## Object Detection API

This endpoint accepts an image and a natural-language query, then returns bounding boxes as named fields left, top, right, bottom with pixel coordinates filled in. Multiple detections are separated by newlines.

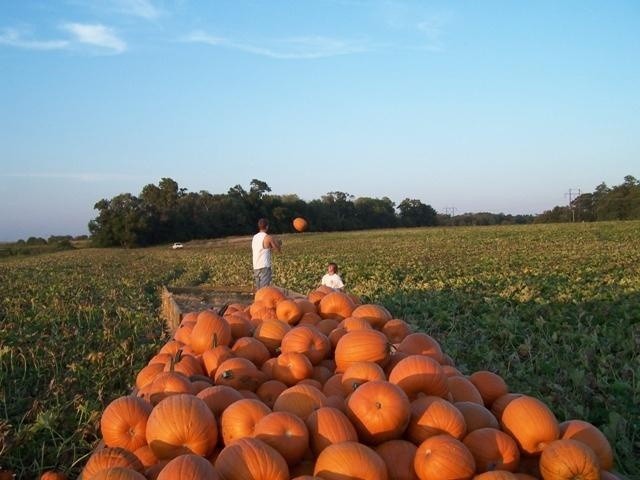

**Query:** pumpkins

left=81, top=285, right=618, bottom=480
left=41, top=468, right=68, bottom=480
left=292, top=217, right=308, bottom=232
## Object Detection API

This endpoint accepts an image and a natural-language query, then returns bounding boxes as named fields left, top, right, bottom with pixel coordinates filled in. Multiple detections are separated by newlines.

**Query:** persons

left=252, top=217, right=281, bottom=289
left=322, top=262, right=345, bottom=293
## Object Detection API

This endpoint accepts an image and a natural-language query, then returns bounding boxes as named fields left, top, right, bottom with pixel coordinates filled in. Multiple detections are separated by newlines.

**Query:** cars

left=172, top=242, right=183, bottom=250
